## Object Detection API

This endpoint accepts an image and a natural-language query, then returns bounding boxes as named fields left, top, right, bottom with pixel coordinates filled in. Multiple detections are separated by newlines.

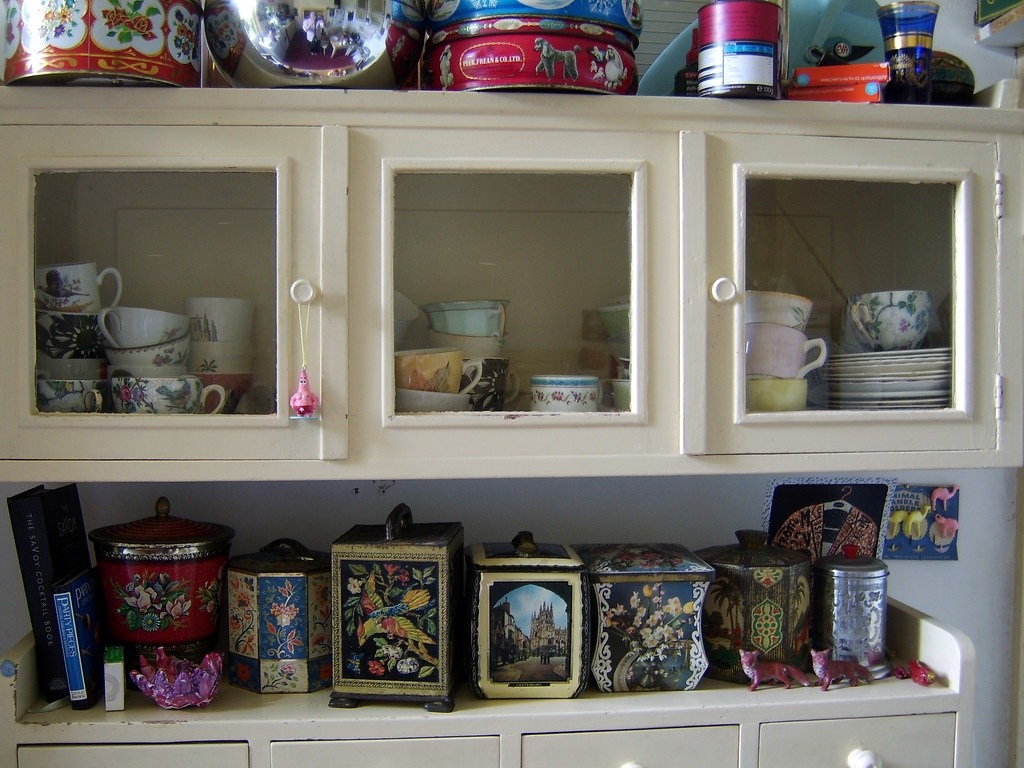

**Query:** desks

left=325, top=27, right=355, bottom=58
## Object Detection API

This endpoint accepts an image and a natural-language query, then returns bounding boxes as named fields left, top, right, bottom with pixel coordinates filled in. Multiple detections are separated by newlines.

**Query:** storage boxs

left=786, top=62, right=891, bottom=103
left=102, top=646, right=125, bottom=711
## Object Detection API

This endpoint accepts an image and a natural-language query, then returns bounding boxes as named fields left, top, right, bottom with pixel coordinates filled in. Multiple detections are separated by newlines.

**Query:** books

left=6, top=482, right=106, bottom=710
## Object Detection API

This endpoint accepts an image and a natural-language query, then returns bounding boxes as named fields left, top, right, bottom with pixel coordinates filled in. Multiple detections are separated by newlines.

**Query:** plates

left=809, top=348, right=952, bottom=409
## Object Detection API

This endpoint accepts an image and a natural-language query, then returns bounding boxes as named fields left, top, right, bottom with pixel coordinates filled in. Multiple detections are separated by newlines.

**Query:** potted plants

left=264, top=7, right=282, bottom=39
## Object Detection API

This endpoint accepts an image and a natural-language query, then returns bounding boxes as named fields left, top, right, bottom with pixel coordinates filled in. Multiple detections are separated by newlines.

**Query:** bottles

left=89, top=495, right=234, bottom=691
left=694, top=528, right=814, bottom=682
left=819, top=545, right=892, bottom=679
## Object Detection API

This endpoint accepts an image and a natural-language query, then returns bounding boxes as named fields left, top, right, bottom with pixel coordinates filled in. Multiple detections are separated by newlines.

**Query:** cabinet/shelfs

left=0, top=595, right=977, bottom=768
left=0, top=86, right=1024, bottom=482
left=287, top=21, right=296, bottom=41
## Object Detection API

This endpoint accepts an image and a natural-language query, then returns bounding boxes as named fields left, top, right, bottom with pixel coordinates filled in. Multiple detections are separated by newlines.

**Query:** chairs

left=320, top=40, right=329, bottom=55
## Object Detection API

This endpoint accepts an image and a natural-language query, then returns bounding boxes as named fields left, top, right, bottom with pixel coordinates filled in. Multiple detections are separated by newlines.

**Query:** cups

left=529, top=294, right=631, bottom=413
left=847, top=290, right=933, bottom=351
left=393, top=298, right=520, bottom=411
left=878, top=3, right=941, bottom=103
left=745, top=289, right=828, bottom=411
left=36, top=260, right=256, bottom=414
